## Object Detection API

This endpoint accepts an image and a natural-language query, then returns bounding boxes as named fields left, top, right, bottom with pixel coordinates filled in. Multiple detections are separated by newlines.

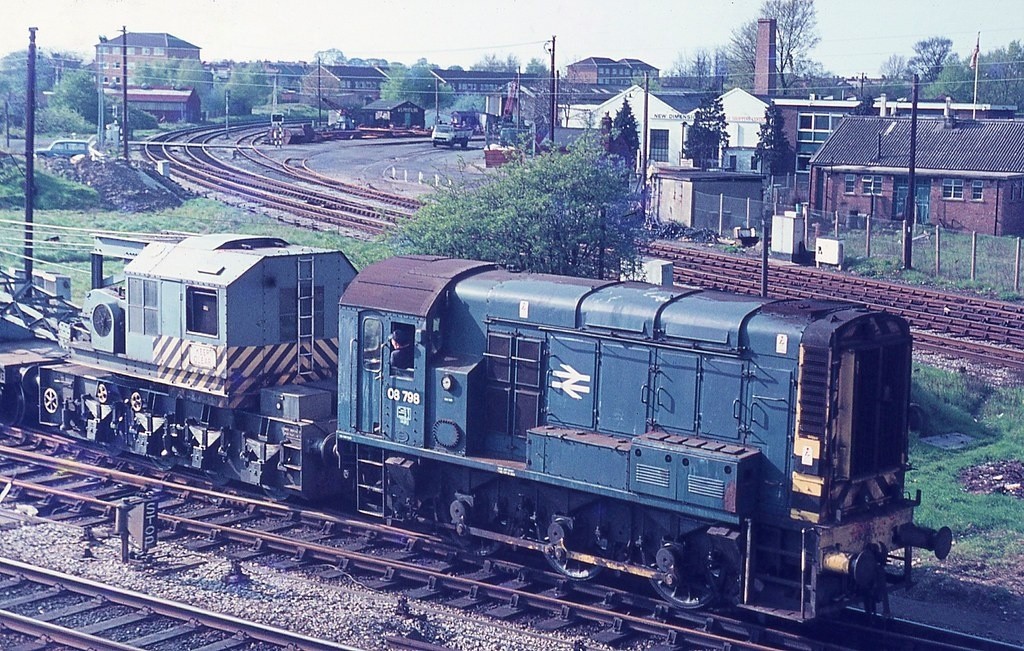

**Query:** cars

left=34, top=139, right=91, bottom=161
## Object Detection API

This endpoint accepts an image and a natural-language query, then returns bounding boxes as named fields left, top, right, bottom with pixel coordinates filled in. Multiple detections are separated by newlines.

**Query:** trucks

left=431, top=124, right=476, bottom=150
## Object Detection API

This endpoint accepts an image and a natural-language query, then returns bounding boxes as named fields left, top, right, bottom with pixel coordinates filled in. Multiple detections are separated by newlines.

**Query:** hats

left=388, top=330, right=409, bottom=344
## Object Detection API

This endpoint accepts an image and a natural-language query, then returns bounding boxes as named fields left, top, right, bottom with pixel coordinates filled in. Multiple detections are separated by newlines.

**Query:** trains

left=2, top=227, right=951, bottom=633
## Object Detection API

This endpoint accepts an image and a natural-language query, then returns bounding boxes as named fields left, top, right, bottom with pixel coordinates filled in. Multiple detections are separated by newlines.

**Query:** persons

left=387, top=329, right=415, bottom=368
